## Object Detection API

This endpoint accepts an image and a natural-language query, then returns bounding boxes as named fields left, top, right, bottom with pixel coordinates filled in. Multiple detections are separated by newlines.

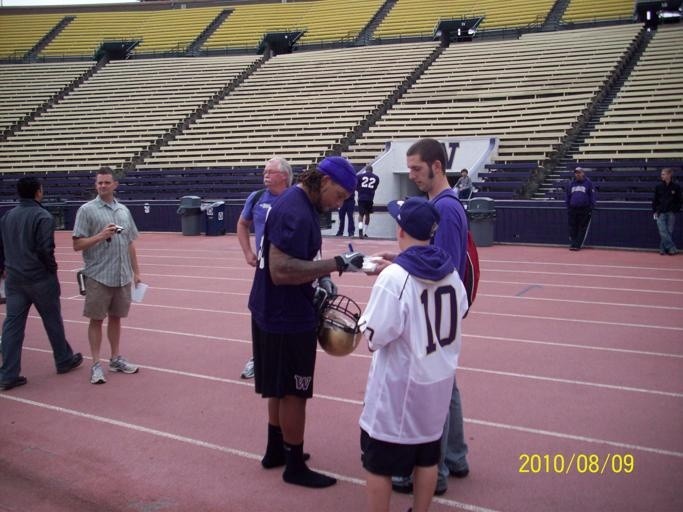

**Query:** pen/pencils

left=348, top=244, right=354, bottom=252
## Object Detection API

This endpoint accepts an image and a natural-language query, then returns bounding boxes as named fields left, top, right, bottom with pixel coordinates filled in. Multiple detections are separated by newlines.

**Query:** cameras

left=113, top=225, right=124, bottom=234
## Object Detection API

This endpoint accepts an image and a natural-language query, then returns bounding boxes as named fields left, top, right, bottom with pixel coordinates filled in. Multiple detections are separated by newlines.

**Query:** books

left=358, top=255, right=384, bottom=273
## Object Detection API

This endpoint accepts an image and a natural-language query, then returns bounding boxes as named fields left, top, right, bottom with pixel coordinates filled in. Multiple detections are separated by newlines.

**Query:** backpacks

left=432, top=194, right=480, bottom=319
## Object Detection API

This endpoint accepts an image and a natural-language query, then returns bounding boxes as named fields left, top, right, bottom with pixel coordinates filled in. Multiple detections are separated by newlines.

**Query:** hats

left=574, top=167, right=583, bottom=173
left=315, top=155, right=358, bottom=194
left=387, top=196, right=440, bottom=240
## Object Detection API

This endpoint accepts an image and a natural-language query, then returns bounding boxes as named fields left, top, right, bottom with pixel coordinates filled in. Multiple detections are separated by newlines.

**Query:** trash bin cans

left=180, top=196, right=200, bottom=235
left=204, top=201, right=226, bottom=236
left=468, top=199, right=497, bottom=248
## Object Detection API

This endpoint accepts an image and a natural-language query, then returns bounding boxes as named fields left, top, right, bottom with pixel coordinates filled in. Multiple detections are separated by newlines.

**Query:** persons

left=0, top=176, right=84, bottom=391
left=563, top=166, right=598, bottom=251
left=363, top=138, right=470, bottom=496
left=652, top=167, right=683, bottom=255
left=355, top=195, right=470, bottom=512
left=72, top=166, right=144, bottom=385
left=334, top=191, right=357, bottom=238
left=239, top=156, right=294, bottom=380
left=455, top=168, right=474, bottom=211
left=354, top=164, right=379, bottom=239
left=246, top=155, right=364, bottom=489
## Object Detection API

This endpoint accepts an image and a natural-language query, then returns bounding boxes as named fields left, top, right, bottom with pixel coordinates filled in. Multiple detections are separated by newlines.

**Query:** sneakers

left=660, top=250, right=665, bottom=255
left=336, top=232, right=343, bottom=236
left=349, top=232, right=353, bottom=239
left=570, top=242, right=579, bottom=250
left=668, top=250, right=679, bottom=254
left=240, top=356, right=255, bottom=379
left=89, top=361, right=107, bottom=384
left=108, top=355, right=139, bottom=374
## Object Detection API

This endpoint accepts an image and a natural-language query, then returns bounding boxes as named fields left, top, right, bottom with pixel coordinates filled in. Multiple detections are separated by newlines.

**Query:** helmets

left=318, top=294, right=362, bottom=357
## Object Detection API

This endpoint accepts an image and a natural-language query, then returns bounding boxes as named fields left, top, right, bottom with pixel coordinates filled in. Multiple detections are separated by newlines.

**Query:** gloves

left=334, top=251, right=364, bottom=277
left=319, top=274, right=337, bottom=301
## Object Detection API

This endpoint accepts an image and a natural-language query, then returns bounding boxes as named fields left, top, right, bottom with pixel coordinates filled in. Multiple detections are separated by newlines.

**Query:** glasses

left=263, top=170, right=279, bottom=174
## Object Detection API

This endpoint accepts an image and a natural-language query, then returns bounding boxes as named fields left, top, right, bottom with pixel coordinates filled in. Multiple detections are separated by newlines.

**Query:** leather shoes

left=0, top=376, right=27, bottom=390
left=391, top=476, right=448, bottom=495
left=57, top=352, right=82, bottom=374
left=449, top=468, right=469, bottom=477
